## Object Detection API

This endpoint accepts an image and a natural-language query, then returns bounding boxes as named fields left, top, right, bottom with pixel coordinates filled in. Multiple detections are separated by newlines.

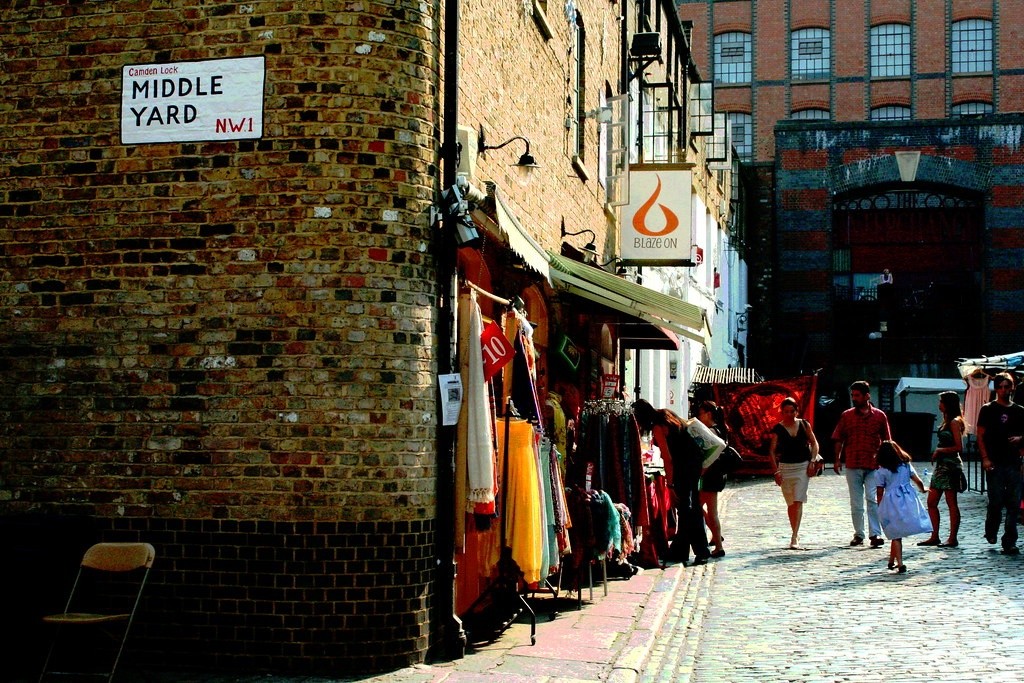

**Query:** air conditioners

left=630, top=32, right=661, bottom=56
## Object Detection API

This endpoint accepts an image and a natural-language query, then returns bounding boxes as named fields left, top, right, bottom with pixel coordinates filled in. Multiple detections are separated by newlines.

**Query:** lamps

left=481, top=137, right=541, bottom=187
left=561, top=221, right=600, bottom=264
left=594, top=254, right=631, bottom=278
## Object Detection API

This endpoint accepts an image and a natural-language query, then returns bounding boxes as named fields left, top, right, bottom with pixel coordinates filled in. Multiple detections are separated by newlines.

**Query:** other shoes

left=1000, top=540, right=1020, bottom=556
left=984, top=534, right=997, bottom=545
left=898, top=565, right=907, bottom=574
left=708, top=536, right=724, bottom=546
left=789, top=535, right=800, bottom=550
left=938, top=539, right=959, bottom=547
left=694, top=554, right=707, bottom=564
left=888, top=562, right=895, bottom=570
left=917, top=538, right=941, bottom=547
left=850, top=536, right=864, bottom=546
left=710, top=550, right=725, bottom=558
left=869, top=535, right=885, bottom=547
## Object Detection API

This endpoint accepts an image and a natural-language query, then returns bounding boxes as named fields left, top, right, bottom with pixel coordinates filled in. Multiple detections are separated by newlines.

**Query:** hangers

left=962, top=363, right=994, bottom=380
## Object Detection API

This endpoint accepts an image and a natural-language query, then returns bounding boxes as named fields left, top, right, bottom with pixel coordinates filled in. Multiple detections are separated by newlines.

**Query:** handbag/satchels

left=806, top=454, right=825, bottom=478
left=705, top=426, right=743, bottom=478
left=679, top=417, right=728, bottom=468
left=953, top=470, right=967, bottom=493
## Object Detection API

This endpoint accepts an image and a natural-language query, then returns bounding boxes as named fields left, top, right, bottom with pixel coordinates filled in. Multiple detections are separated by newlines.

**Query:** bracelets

left=774, top=470, right=781, bottom=475
left=810, top=458, right=816, bottom=463
left=666, top=484, right=675, bottom=489
left=982, top=454, right=988, bottom=458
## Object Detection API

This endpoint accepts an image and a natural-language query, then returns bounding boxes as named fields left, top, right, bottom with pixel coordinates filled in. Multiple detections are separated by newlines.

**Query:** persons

left=770, top=397, right=819, bottom=550
left=916, top=391, right=967, bottom=547
left=976, top=372, right=1024, bottom=554
left=630, top=399, right=727, bottom=566
left=832, top=381, right=892, bottom=547
left=873, top=441, right=930, bottom=572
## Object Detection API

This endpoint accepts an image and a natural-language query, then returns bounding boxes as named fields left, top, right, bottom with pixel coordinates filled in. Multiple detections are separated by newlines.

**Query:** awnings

left=493, top=184, right=553, bottom=289
left=546, top=250, right=712, bottom=347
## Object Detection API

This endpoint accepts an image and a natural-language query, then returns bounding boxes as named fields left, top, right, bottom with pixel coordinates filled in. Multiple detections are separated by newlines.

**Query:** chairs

left=41, top=542, right=156, bottom=683
